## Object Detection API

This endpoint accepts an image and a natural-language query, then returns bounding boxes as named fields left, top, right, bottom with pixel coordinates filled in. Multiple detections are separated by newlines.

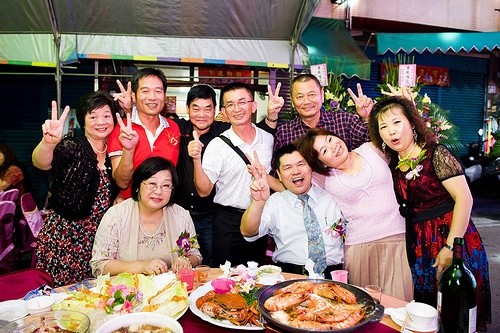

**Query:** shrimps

left=265, top=280, right=366, bottom=330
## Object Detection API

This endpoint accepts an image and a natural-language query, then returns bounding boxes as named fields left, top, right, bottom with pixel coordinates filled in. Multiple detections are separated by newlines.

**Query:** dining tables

left=52, top=268, right=438, bottom=333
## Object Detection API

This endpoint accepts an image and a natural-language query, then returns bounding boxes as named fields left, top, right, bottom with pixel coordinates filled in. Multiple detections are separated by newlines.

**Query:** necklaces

left=396, top=140, right=418, bottom=162
left=87, top=138, right=108, bottom=152
left=139, top=216, right=164, bottom=237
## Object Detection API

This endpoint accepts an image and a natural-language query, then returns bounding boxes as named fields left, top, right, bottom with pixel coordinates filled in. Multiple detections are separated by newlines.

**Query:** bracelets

left=266, top=116, right=279, bottom=123
left=444, top=243, right=454, bottom=251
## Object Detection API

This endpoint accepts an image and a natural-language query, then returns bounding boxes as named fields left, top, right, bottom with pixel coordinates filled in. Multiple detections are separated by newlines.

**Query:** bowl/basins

left=90, top=311, right=184, bottom=333
left=25, top=295, right=54, bottom=313
left=256, top=278, right=385, bottom=332
left=405, top=301, right=437, bottom=326
left=0, top=310, right=91, bottom=333
left=211, top=279, right=237, bottom=293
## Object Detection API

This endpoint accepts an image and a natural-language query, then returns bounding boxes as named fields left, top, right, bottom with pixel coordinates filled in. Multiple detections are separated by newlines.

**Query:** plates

left=391, top=307, right=439, bottom=332
left=0, top=299, right=28, bottom=324
left=57, top=269, right=271, bottom=333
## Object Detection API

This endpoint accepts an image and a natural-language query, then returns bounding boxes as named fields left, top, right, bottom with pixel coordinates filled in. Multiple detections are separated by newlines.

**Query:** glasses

left=141, top=179, right=174, bottom=192
left=223, top=100, right=254, bottom=107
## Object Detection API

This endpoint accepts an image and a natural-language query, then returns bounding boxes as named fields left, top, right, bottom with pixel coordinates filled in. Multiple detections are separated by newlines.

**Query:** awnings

left=368, top=26, right=500, bottom=60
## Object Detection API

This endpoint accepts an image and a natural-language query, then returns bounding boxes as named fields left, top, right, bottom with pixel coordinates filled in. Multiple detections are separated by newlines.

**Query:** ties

left=298, top=194, right=327, bottom=276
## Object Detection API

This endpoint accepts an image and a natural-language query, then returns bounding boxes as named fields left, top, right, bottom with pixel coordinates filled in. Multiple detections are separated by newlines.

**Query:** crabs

left=195, top=289, right=262, bottom=328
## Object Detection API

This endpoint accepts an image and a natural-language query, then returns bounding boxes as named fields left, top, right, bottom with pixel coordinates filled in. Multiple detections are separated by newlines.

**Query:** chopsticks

left=401, top=311, right=411, bottom=332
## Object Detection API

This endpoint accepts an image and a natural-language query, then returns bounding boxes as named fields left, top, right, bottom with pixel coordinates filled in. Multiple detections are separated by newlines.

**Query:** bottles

left=437, top=236, right=477, bottom=333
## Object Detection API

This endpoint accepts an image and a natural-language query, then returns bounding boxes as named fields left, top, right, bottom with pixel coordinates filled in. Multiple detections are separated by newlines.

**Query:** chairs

left=0, top=187, right=19, bottom=201
left=0, top=271, right=54, bottom=303
left=0, top=201, right=16, bottom=243
left=18, top=191, right=46, bottom=238
left=0, top=242, right=15, bottom=270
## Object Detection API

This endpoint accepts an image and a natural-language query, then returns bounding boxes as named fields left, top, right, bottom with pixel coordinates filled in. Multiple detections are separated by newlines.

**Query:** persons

left=369, top=94, right=492, bottom=332
left=247, top=82, right=416, bottom=301
left=106, top=67, right=182, bottom=202
left=269, top=73, right=375, bottom=185
left=88, top=155, right=203, bottom=275
left=187, top=82, right=274, bottom=267
left=239, top=142, right=347, bottom=281
left=110, top=78, right=286, bottom=265
left=166, top=112, right=179, bottom=120
left=0, top=143, right=27, bottom=221
left=31, top=90, right=117, bottom=286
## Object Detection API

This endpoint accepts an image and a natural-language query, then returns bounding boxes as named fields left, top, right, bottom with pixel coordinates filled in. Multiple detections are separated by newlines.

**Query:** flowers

left=173, top=231, right=203, bottom=258
left=372, top=68, right=464, bottom=152
left=395, top=148, right=428, bottom=180
left=324, top=215, right=349, bottom=241
left=93, top=281, right=140, bottom=315
left=320, top=71, right=355, bottom=111
left=210, top=260, right=260, bottom=295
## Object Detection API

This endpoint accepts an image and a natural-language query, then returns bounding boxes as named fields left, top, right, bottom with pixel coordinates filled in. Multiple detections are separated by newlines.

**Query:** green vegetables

left=237, top=286, right=261, bottom=305
left=104, top=290, right=136, bottom=314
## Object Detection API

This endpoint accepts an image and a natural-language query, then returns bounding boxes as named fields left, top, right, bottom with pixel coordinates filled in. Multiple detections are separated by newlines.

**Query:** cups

left=179, top=268, right=195, bottom=292
left=195, top=265, right=210, bottom=287
left=364, top=284, right=382, bottom=303
left=330, top=270, right=349, bottom=284
left=258, top=264, right=282, bottom=285
